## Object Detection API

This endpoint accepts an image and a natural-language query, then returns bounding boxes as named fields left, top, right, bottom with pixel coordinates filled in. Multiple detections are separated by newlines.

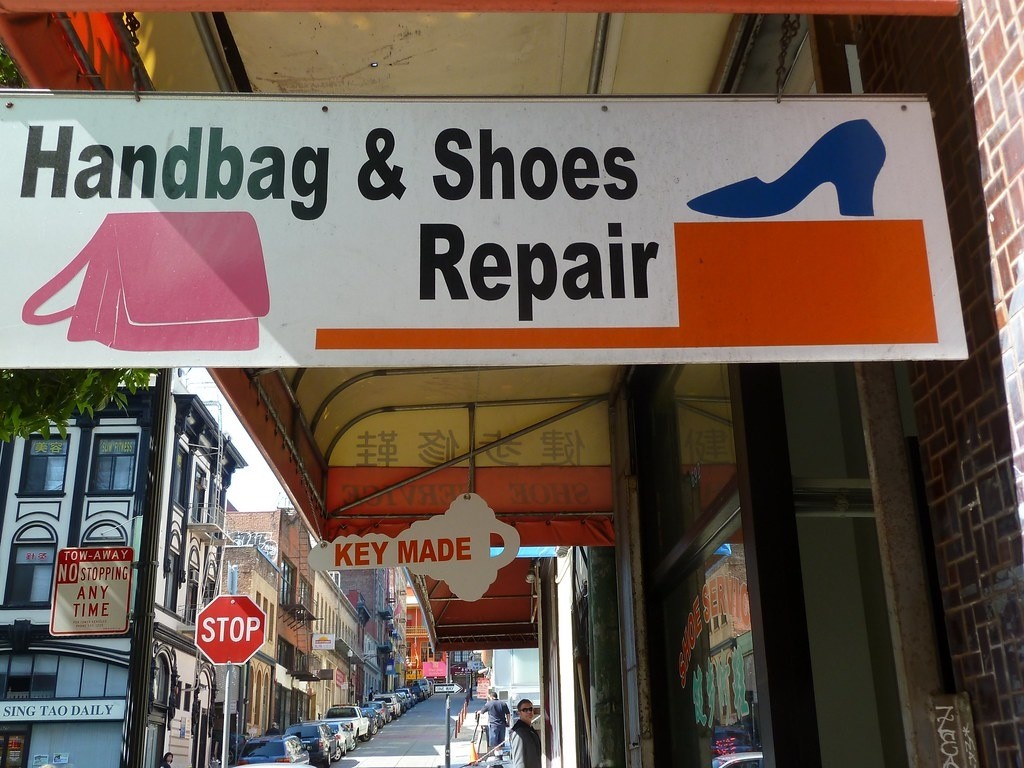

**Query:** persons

left=266, top=722, right=281, bottom=736
left=161, top=752, right=173, bottom=768
left=369, top=687, right=380, bottom=701
left=510, top=699, right=541, bottom=768
left=478, top=692, right=510, bottom=768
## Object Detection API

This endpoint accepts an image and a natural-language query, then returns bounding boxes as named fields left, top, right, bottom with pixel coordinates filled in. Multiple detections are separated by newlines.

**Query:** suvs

left=237, top=733, right=313, bottom=765
left=284, top=721, right=342, bottom=768
left=320, top=703, right=372, bottom=745
left=372, top=691, right=403, bottom=720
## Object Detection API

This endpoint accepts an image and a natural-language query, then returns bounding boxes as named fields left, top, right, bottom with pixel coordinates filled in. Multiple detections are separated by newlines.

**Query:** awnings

left=281, top=604, right=316, bottom=622
left=288, top=671, right=320, bottom=682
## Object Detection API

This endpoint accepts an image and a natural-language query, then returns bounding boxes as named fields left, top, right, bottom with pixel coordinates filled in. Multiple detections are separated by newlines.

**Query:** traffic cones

left=469, top=741, right=481, bottom=765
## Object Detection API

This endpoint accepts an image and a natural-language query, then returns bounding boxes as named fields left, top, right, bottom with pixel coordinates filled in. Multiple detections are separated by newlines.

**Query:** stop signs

left=194, top=595, right=267, bottom=665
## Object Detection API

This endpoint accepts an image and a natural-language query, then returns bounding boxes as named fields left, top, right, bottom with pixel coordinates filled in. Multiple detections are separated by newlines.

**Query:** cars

left=360, top=707, right=385, bottom=735
left=361, top=701, right=393, bottom=725
left=392, top=678, right=432, bottom=715
left=711, top=724, right=763, bottom=768
left=327, top=721, right=356, bottom=756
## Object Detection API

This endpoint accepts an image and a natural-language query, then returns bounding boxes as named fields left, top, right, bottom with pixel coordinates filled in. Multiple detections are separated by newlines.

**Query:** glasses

left=520, top=707, right=533, bottom=712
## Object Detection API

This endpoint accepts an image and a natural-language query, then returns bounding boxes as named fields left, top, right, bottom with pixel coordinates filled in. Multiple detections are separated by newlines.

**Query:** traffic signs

left=433, top=682, right=463, bottom=696
